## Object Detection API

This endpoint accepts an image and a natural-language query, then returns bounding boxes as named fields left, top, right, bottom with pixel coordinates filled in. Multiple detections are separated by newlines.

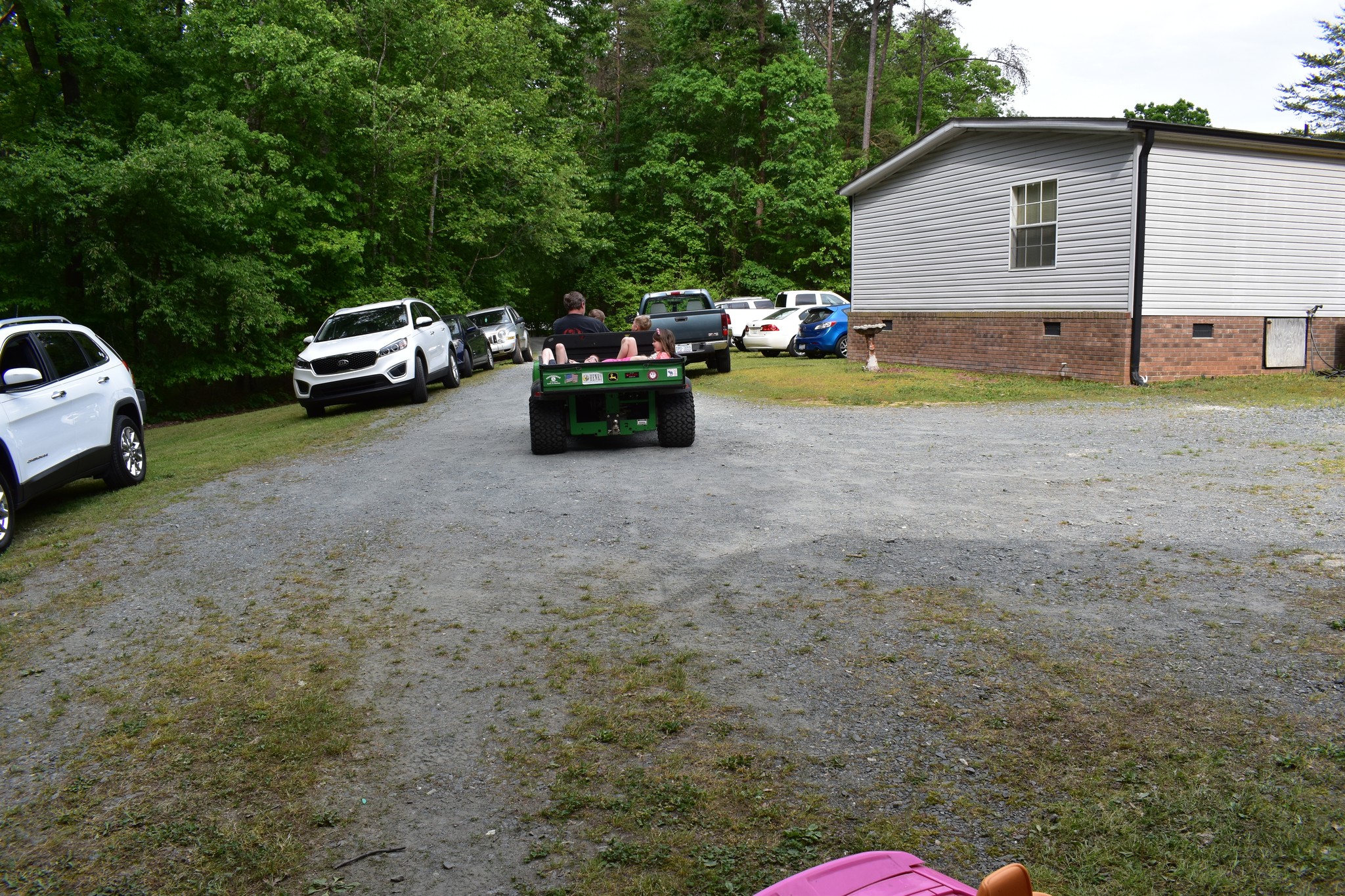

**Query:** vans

left=725, top=290, right=851, bottom=352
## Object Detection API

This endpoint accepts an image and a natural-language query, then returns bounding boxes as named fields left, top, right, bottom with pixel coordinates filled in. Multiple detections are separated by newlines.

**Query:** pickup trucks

left=625, top=288, right=731, bottom=373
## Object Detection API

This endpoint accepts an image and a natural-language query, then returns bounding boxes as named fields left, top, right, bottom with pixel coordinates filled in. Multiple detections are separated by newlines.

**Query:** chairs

left=0, top=344, right=38, bottom=382
left=686, top=299, right=703, bottom=311
left=650, top=302, right=667, bottom=314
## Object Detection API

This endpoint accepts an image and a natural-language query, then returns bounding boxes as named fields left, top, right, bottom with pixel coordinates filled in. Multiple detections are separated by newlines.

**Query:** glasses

left=585, top=357, right=598, bottom=362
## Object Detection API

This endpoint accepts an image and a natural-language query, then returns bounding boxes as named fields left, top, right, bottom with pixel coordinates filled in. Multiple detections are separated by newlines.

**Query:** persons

left=601, top=328, right=676, bottom=362
left=542, top=343, right=601, bottom=365
left=589, top=309, right=605, bottom=323
left=632, top=315, right=651, bottom=332
left=553, top=292, right=611, bottom=335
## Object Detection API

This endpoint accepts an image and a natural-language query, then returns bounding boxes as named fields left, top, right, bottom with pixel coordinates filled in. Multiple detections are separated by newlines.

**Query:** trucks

left=528, top=326, right=696, bottom=456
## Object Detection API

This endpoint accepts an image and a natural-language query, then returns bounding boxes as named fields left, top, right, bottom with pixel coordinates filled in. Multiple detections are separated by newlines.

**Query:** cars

left=793, top=303, right=851, bottom=359
left=440, top=314, right=494, bottom=378
left=742, top=307, right=814, bottom=357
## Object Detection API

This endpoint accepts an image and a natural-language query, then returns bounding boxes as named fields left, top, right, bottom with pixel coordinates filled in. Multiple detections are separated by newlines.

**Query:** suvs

left=292, top=298, right=460, bottom=418
left=713, top=296, right=775, bottom=347
left=466, top=305, right=533, bottom=365
left=0, top=314, right=148, bottom=555
